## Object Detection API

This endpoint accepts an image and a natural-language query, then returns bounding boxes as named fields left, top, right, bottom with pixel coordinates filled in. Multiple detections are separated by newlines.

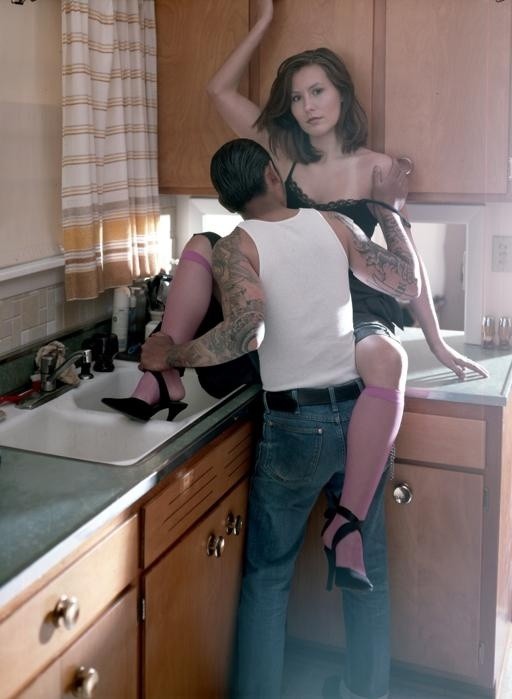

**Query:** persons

left=139, top=138, right=421, bottom=696
left=99, top=1, right=490, bottom=594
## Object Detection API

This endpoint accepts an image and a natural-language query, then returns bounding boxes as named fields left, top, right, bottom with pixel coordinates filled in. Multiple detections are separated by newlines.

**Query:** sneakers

left=321, top=675, right=344, bottom=699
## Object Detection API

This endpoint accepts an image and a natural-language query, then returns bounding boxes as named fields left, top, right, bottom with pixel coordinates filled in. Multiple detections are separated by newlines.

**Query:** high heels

left=100, top=368, right=190, bottom=423
left=318, top=503, right=375, bottom=594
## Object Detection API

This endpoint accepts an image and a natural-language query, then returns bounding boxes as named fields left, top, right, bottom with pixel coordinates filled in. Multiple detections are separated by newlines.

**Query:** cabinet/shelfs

left=290, top=405, right=510, bottom=695
left=0, top=508, right=140, bottom=699
left=144, top=418, right=262, bottom=695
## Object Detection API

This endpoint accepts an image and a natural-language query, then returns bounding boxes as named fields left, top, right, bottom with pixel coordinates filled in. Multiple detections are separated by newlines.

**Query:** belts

left=259, top=376, right=367, bottom=415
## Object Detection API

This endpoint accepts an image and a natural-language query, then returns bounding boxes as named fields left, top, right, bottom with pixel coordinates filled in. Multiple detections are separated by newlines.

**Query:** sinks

left=0, top=360, right=246, bottom=429
left=0, top=402, right=182, bottom=466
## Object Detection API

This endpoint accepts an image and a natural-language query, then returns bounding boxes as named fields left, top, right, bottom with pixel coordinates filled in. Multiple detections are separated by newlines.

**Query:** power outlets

left=490, top=233, right=512, bottom=273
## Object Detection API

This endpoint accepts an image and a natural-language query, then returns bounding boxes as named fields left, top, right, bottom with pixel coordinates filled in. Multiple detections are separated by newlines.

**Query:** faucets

left=39, top=350, right=91, bottom=391
